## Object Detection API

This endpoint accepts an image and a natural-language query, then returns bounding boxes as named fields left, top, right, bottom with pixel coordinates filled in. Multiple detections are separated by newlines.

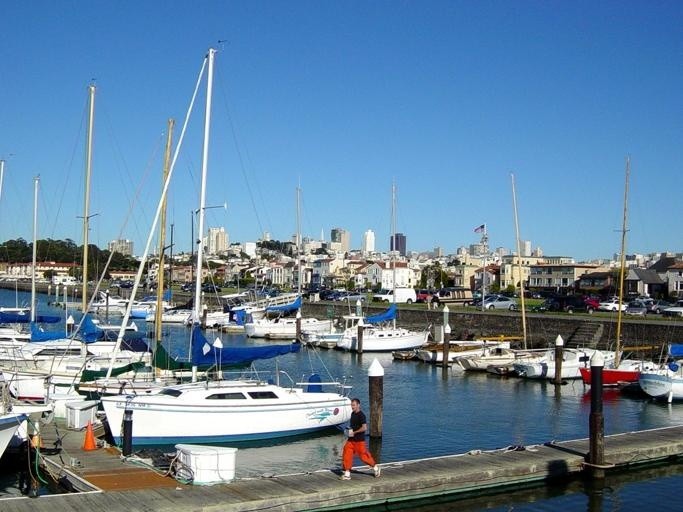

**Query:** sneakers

left=374, top=465, right=381, bottom=478
left=338, top=475, right=351, bottom=480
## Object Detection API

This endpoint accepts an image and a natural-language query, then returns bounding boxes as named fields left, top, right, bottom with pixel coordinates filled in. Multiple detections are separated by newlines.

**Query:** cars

left=302, top=289, right=366, bottom=303
left=532, top=290, right=683, bottom=318
left=463, top=296, right=518, bottom=312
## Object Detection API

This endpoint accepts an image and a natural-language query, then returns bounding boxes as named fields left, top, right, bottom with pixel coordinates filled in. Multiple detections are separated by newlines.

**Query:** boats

left=639, top=357, right=683, bottom=404
left=98, top=368, right=357, bottom=451
left=392, top=338, right=511, bottom=363
left=486, top=347, right=624, bottom=381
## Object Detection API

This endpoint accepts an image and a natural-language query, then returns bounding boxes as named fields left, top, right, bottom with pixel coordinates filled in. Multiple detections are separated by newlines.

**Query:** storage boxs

left=47, top=395, right=83, bottom=418
left=175, top=444, right=238, bottom=485
left=66, top=403, right=99, bottom=429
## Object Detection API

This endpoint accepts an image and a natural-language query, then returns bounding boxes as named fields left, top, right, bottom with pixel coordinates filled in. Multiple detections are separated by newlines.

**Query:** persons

left=336, top=398, right=382, bottom=480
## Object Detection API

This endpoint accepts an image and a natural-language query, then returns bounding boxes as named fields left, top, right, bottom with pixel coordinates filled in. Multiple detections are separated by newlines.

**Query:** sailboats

left=337, top=179, right=430, bottom=351
left=0, top=47, right=354, bottom=419
left=578, top=155, right=649, bottom=388
left=453, top=175, right=552, bottom=371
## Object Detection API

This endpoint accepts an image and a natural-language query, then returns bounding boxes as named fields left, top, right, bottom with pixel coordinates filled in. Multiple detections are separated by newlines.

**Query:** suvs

left=416, top=289, right=434, bottom=304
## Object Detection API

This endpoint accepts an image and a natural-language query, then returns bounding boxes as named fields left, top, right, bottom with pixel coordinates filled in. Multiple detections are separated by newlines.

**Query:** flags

left=473, top=224, right=484, bottom=233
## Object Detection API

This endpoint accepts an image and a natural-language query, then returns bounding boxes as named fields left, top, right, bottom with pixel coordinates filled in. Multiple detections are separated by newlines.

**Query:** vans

left=372, top=288, right=417, bottom=305
